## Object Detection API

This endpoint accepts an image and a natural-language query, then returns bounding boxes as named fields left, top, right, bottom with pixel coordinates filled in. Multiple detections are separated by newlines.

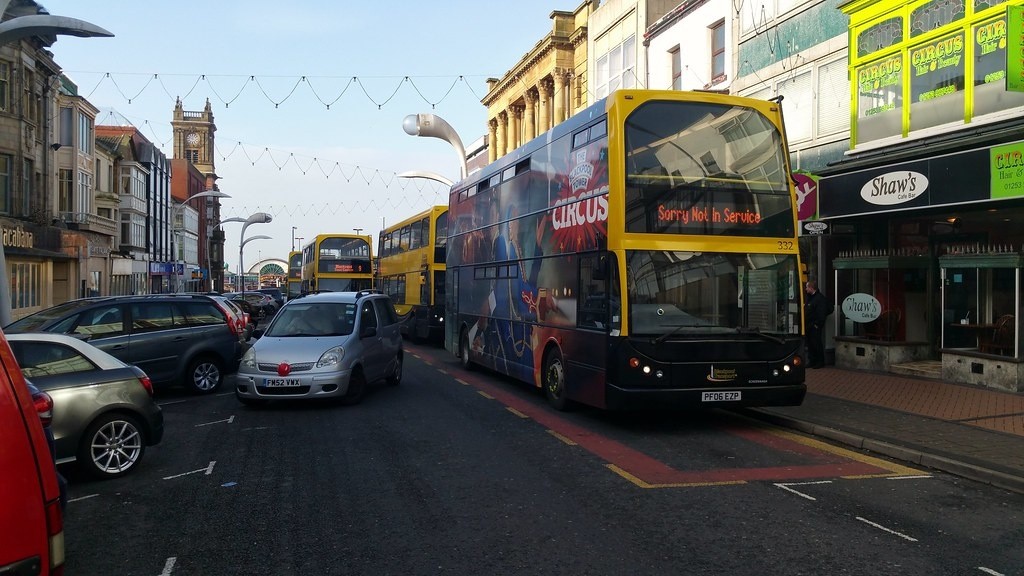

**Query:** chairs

left=863, top=308, right=901, bottom=341
left=97, top=312, right=117, bottom=325
left=980, top=314, right=1015, bottom=356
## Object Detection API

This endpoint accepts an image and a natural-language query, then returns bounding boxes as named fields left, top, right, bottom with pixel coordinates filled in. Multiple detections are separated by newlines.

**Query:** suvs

left=258, top=289, right=285, bottom=305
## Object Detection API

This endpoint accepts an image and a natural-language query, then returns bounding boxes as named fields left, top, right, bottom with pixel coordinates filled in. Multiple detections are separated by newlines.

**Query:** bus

left=301, top=234, right=375, bottom=294
left=444, top=88, right=807, bottom=415
left=286, top=252, right=302, bottom=301
left=376, top=205, right=448, bottom=341
left=371, top=255, right=378, bottom=292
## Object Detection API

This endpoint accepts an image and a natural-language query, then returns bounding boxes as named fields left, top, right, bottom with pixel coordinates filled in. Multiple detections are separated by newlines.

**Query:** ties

left=515, top=240, right=526, bottom=283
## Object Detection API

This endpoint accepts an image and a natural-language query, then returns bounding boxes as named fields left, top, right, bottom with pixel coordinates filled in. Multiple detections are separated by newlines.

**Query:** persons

left=803, top=281, right=834, bottom=369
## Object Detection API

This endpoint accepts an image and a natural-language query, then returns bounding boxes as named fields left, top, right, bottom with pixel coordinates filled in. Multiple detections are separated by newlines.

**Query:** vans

left=0, top=328, right=66, bottom=576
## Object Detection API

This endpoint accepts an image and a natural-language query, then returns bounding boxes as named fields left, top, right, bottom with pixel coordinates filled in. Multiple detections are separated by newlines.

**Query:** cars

left=0, top=326, right=164, bottom=512
left=2, top=296, right=242, bottom=394
left=211, top=292, right=280, bottom=343
left=236, top=291, right=404, bottom=406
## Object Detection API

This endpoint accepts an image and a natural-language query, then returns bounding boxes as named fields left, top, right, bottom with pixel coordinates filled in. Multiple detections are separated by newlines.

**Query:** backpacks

left=820, top=292, right=834, bottom=316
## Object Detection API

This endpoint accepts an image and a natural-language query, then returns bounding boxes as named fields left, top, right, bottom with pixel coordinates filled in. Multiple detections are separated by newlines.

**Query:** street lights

left=239, top=212, right=273, bottom=291
left=295, top=238, right=304, bottom=251
left=172, top=190, right=232, bottom=292
left=206, top=215, right=247, bottom=290
left=353, top=229, right=363, bottom=234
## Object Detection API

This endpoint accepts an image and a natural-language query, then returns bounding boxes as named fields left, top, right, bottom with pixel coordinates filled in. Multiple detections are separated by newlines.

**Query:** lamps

left=50, top=143, right=62, bottom=151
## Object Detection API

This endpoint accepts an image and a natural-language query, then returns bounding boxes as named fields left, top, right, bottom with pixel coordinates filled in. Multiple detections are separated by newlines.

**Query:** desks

left=949, top=321, right=998, bottom=351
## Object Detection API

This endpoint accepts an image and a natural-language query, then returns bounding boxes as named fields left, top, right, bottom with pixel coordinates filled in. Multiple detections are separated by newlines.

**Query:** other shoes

left=805, top=363, right=814, bottom=368
left=813, top=363, right=826, bottom=369
